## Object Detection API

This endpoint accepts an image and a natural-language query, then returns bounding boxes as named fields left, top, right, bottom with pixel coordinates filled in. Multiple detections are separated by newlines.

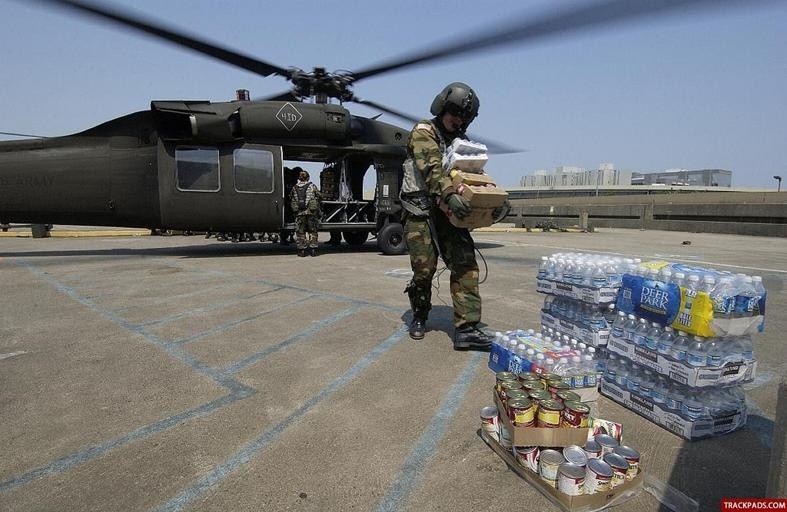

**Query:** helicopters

left=1, top=0, right=761, bottom=253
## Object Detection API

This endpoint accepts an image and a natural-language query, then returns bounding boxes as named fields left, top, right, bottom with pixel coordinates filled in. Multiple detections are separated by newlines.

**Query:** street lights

left=772, top=175, right=781, bottom=193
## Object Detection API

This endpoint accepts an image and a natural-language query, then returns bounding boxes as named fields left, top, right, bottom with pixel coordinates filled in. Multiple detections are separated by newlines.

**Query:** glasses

left=445, top=103, right=474, bottom=126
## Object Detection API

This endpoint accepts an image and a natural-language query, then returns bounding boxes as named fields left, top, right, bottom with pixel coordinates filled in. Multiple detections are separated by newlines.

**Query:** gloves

left=440, top=185, right=472, bottom=221
left=491, top=199, right=512, bottom=224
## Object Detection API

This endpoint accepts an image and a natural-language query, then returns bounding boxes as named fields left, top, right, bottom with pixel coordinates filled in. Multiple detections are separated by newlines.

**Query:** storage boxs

left=441, top=169, right=508, bottom=229
left=475, top=371, right=645, bottom=512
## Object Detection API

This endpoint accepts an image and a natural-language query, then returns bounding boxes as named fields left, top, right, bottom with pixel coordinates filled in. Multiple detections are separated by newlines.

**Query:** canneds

left=478, top=406, right=639, bottom=495
left=493, top=370, right=587, bottom=430
left=437, top=137, right=496, bottom=220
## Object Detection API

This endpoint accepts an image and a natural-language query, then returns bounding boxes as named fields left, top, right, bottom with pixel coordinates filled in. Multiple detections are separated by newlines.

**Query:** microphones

left=453, top=123, right=466, bottom=134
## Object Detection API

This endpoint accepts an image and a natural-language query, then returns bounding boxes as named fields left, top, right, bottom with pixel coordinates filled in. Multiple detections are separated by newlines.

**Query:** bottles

left=487, top=249, right=767, bottom=443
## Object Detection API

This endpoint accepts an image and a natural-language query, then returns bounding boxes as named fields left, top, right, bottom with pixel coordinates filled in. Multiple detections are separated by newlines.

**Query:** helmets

left=430, top=82, right=480, bottom=134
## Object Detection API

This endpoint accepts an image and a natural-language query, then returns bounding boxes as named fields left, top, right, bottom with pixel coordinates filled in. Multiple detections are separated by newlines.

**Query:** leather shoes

left=408, top=316, right=426, bottom=339
left=297, top=248, right=320, bottom=256
left=179, top=233, right=297, bottom=246
left=454, top=322, right=494, bottom=348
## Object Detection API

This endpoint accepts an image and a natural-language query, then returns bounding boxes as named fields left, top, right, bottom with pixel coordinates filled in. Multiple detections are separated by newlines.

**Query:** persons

left=398, top=81, right=514, bottom=352
left=288, top=170, right=325, bottom=258
left=185, top=229, right=344, bottom=246
